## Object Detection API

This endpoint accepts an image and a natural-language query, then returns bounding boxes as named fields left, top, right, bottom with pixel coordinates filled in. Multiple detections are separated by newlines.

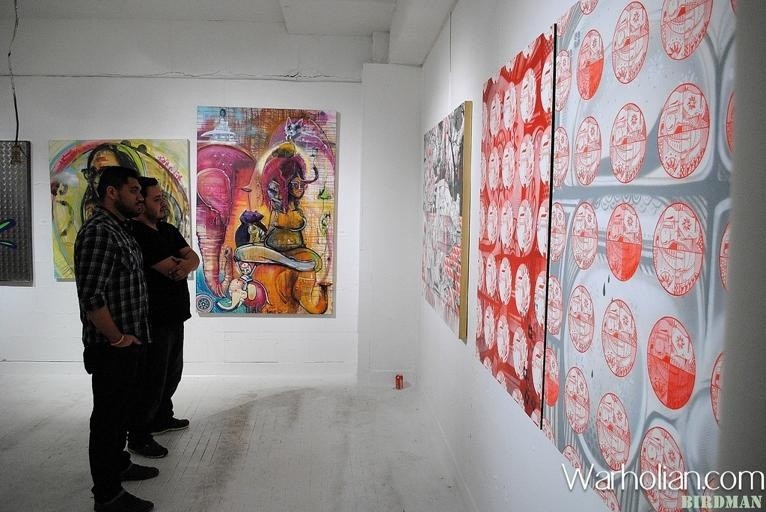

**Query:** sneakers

left=150, top=417, right=189, bottom=435
left=93, top=485, right=154, bottom=512
left=120, top=460, right=160, bottom=483
left=128, top=432, right=168, bottom=460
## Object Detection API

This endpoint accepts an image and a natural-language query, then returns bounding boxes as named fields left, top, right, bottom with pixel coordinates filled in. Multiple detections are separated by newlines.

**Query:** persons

left=73, top=167, right=159, bottom=512
left=127, top=176, right=201, bottom=459
left=79, top=141, right=142, bottom=222
left=235, top=155, right=316, bottom=273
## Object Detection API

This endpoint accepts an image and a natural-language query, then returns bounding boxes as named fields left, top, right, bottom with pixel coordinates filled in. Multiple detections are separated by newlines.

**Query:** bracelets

left=108, top=333, right=126, bottom=347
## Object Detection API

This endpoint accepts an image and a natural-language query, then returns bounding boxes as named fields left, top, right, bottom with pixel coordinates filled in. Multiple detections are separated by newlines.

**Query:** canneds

left=396, top=375, right=403, bottom=389
